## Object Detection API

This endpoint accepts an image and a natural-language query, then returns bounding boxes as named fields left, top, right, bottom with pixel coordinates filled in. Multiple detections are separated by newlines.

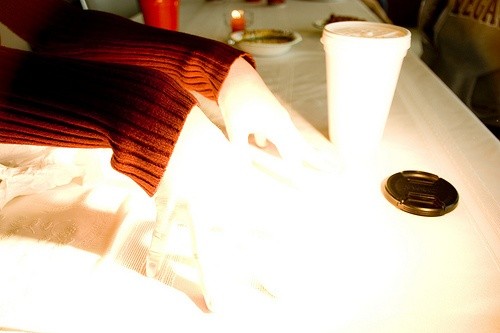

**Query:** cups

left=139, top=0, right=180, bottom=32
left=320, top=19, right=411, bottom=163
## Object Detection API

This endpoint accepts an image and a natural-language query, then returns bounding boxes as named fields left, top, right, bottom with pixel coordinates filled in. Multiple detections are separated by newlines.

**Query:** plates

left=229, top=28, right=304, bottom=56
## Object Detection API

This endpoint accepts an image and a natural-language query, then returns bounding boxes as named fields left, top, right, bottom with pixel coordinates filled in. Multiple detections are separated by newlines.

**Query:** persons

left=0, top=0, right=302, bottom=202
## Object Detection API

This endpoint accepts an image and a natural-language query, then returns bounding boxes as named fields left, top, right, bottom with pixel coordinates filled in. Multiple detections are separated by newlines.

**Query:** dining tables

left=0, top=0, right=500, bottom=333
